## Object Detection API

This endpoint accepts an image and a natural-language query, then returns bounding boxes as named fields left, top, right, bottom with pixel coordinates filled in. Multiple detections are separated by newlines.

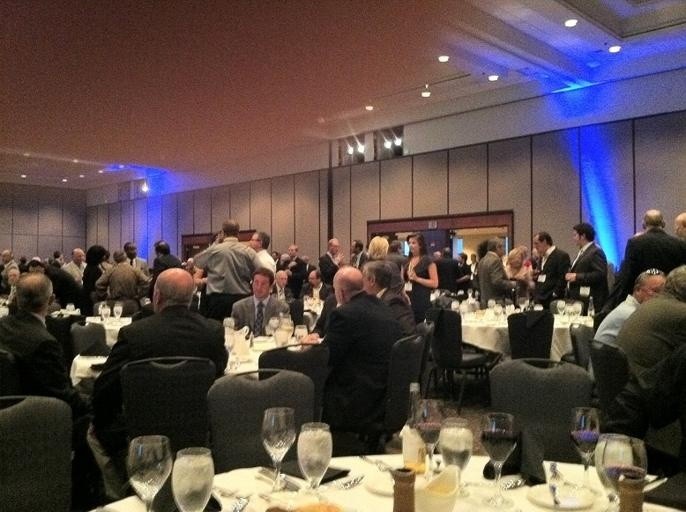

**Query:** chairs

left=588, top=341, right=641, bottom=435
left=485, top=359, right=595, bottom=482
left=426, top=310, right=488, bottom=418
left=1, top=396, right=77, bottom=512
left=508, top=310, right=554, bottom=368
left=383, top=336, right=426, bottom=443
left=209, top=366, right=317, bottom=471
left=568, top=322, right=592, bottom=371
left=257, top=341, right=331, bottom=416
left=122, top=355, right=215, bottom=455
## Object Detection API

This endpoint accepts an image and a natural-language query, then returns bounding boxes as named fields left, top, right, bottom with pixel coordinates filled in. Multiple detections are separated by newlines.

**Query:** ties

left=254, top=302, right=264, bottom=336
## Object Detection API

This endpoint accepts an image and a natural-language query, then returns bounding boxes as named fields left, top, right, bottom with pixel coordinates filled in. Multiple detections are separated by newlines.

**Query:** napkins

left=151, top=475, right=222, bottom=512
left=267, top=459, right=348, bottom=485
left=642, top=473, right=684, bottom=509
left=482, top=429, right=546, bottom=487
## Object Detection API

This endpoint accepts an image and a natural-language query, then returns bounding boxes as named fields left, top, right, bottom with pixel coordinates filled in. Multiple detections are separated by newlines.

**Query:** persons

left=0, top=208, right=686, bottom=476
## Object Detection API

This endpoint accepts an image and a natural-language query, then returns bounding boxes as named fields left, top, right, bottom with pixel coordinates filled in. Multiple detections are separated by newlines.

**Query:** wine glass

left=262, top=408, right=333, bottom=503
left=269, top=317, right=279, bottom=338
left=127, top=434, right=173, bottom=512
left=413, top=399, right=518, bottom=509
left=448, top=299, right=582, bottom=326
left=102, top=305, right=123, bottom=326
left=569, top=406, right=647, bottom=511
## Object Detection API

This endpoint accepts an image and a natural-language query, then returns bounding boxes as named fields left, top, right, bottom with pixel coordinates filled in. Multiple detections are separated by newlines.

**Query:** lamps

left=421, top=82, right=431, bottom=97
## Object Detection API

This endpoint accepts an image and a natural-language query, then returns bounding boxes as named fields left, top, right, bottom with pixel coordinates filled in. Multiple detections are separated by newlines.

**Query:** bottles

left=402, top=382, right=426, bottom=474
left=587, top=296, right=595, bottom=319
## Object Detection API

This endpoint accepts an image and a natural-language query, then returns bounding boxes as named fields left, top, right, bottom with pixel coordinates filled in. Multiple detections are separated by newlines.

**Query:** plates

left=526, top=483, right=596, bottom=510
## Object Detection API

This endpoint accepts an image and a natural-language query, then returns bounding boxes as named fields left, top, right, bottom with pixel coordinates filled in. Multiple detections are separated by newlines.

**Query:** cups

left=295, top=325, right=308, bottom=341
left=274, top=313, right=293, bottom=346
left=174, top=448, right=215, bottom=512
